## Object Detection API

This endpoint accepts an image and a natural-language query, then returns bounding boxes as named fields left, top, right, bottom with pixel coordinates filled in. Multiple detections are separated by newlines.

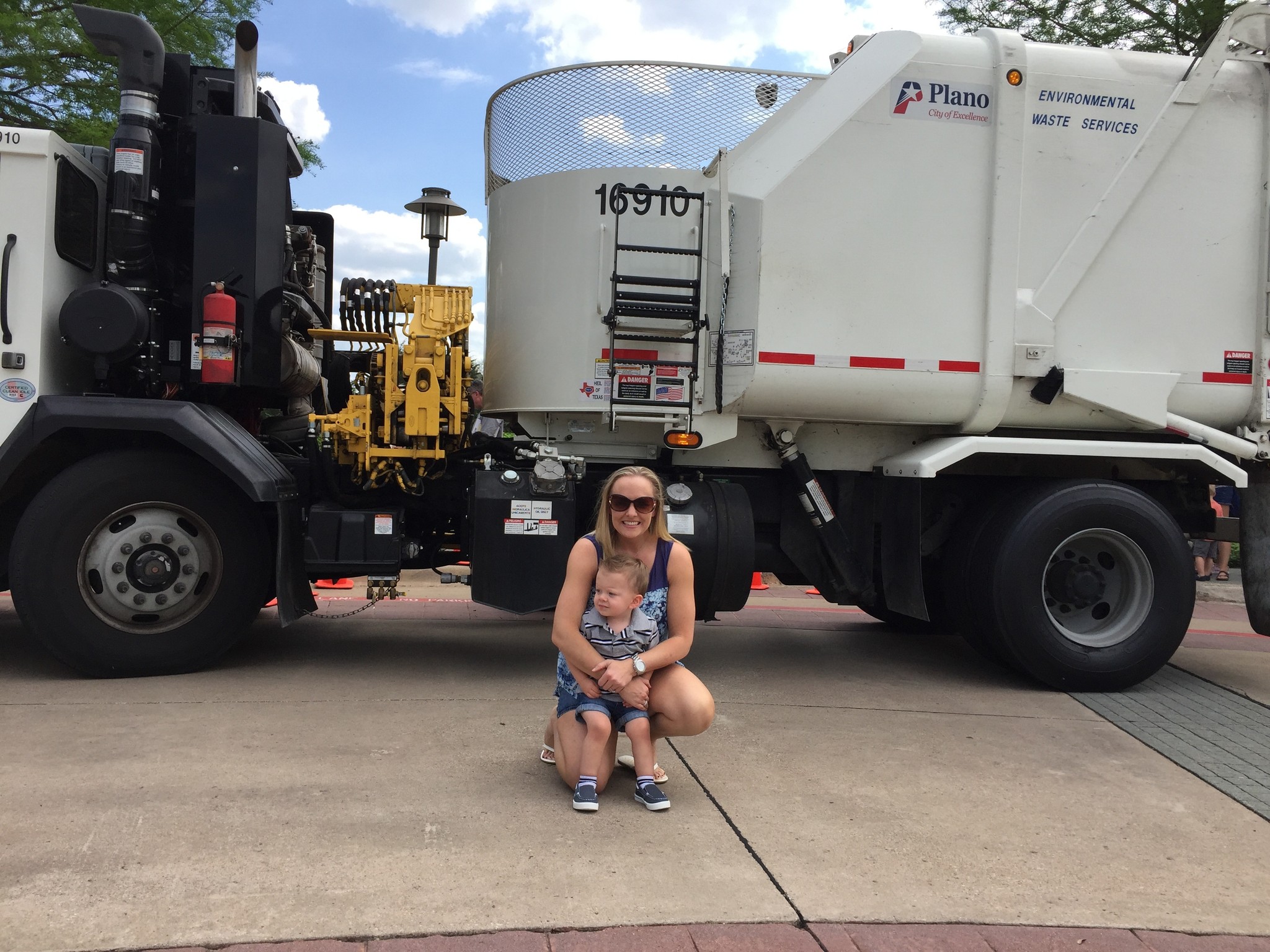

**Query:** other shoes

left=1211, top=566, right=1230, bottom=574
left=1204, top=575, right=1211, bottom=581
left=1196, top=574, right=1206, bottom=582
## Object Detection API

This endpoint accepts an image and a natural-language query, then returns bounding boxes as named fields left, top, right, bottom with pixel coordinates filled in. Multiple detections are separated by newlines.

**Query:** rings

left=609, top=687, right=616, bottom=693
left=643, top=700, right=647, bottom=706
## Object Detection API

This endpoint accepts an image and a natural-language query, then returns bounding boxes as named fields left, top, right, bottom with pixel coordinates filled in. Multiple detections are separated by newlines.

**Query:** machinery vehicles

left=0, top=0, right=1267, bottom=693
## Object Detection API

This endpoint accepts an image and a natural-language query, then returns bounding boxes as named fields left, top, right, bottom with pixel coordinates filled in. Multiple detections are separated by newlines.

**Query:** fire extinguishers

left=194, top=266, right=243, bottom=384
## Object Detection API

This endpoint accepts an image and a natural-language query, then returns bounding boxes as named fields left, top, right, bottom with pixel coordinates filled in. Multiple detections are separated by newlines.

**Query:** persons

left=467, top=379, right=504, bottom=438
left=564, top=553, right=670, bottom=811
left=540, top=466, right=715, bottom=784
left=1186, top=485, right=1235, bottom=581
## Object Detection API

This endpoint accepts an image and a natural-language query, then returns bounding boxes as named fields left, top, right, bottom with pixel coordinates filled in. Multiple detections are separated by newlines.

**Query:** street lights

left=404, top=187, right=468, bottom=285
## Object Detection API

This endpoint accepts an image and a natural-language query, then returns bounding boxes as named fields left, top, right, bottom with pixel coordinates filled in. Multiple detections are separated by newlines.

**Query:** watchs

left=630, top=654, right=647, bottom=675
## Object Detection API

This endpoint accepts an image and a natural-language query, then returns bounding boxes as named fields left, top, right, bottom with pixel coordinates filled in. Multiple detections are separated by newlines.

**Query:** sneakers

left=634, top=783, right=671, bottom=810
left=573, top=782, right=598, bottom=811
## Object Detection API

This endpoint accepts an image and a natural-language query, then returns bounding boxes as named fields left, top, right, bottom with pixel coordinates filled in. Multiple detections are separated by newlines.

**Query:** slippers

left=540, top=744, right=556, bottom=764
left=1216, top=571, right=1229, bottom=581
left=617, top=755, right=668, bottom=784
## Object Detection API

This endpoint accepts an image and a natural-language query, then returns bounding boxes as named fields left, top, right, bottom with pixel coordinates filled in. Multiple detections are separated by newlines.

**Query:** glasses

left=608, top=493, right=658, bottom=514
